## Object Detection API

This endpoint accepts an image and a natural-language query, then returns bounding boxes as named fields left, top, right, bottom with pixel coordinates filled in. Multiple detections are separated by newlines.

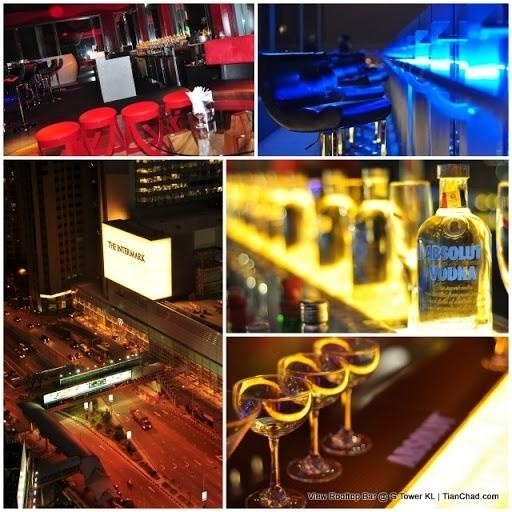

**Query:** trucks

left=132, top=407, right=152, bottom=430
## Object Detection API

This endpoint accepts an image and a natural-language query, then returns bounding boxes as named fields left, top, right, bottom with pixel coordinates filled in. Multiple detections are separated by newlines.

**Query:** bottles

left=226, top=160, right=506, bottom=333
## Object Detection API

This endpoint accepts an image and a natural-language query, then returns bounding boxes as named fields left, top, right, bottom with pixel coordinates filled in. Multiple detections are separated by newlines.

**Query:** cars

left=4, top=310, right=106, bottom=366
left=4, top=411, right=11, bottom=422
left=112, top=484, right=123, bottom=498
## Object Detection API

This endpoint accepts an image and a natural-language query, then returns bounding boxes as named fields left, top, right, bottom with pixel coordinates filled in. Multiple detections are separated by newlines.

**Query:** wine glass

left=232, top=339, right=382, bottom=507
left=186, top=85, right=218, bottom=130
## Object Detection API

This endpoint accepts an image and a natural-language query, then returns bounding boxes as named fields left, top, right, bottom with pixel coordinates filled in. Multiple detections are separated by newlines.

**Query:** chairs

left=5, top=57, right=62, bottom=134
left=200, top=35, right=254, bottom=117
left=258, top=48, right=394, bottom=157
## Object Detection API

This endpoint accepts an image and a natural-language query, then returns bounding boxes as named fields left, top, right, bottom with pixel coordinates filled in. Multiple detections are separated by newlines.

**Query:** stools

left=36, top=87, right=196, bottom=157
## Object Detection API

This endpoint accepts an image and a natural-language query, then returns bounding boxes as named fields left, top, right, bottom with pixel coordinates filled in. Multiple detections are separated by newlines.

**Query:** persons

left=339, top=34, right=355, bottom=52
